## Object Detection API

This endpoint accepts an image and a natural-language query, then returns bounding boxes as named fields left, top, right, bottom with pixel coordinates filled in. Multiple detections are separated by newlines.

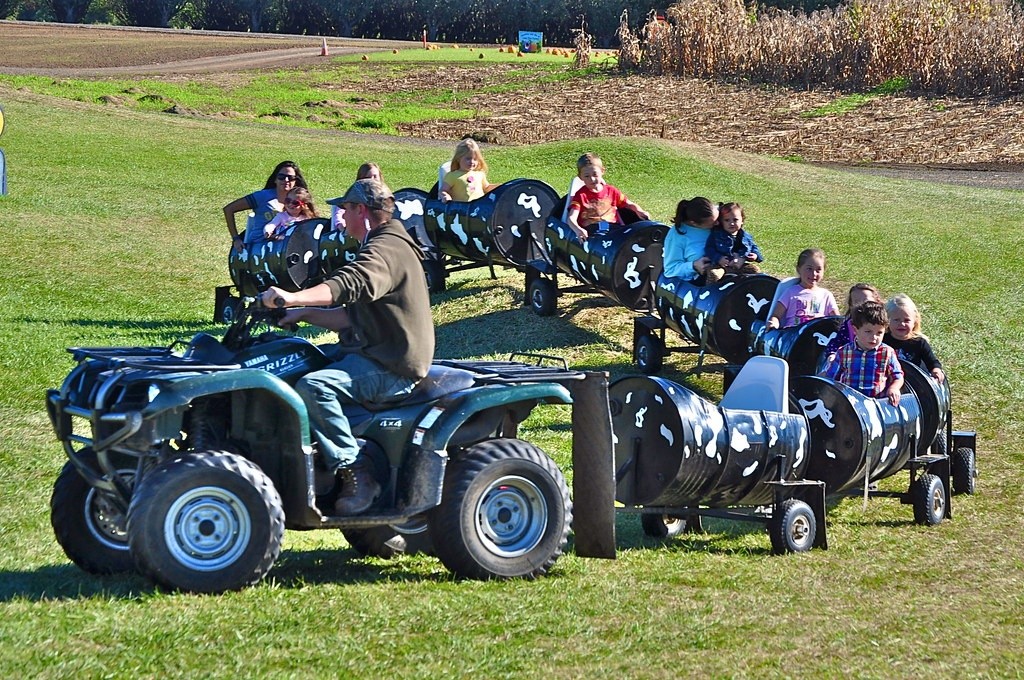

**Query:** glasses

left=284, top=198, right=304, bottom=206
left=277, top=173, right=296, bottom=182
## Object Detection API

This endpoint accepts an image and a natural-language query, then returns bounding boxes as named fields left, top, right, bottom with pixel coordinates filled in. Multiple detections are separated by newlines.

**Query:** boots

left=334, top=467, right=382, bottom=516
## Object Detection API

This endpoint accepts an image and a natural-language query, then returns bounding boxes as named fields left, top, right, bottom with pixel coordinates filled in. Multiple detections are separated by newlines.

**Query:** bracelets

left=233, top=235, right=241, bottom=241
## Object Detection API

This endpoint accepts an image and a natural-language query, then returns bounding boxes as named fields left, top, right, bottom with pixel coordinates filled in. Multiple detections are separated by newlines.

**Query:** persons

left=816, top=283, right=945, bottom=407
left=261, top=178, right=436, bottom=515
left=439, top=138, right=498, bottom=202
left=663, top=197, right=763, bottom=286
left=223, top=161, right=316, bottom=253
left=766, top=248, right=840, bottom=332
left=567, top=153, right=649, bottom=245
left=334, top=162, right=383, bottom=234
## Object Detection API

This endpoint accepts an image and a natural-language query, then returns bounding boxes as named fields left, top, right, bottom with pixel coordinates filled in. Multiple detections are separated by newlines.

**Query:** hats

left=326, top=179, right=396, bottom=212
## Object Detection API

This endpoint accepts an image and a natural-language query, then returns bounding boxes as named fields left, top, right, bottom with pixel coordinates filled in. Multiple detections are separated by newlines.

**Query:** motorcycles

left=46, top=296, right=585, bottom=596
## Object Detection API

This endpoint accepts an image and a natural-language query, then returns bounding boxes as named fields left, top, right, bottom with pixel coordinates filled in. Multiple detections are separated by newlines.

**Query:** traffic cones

left=321, top=37, right=328, bottom=55
